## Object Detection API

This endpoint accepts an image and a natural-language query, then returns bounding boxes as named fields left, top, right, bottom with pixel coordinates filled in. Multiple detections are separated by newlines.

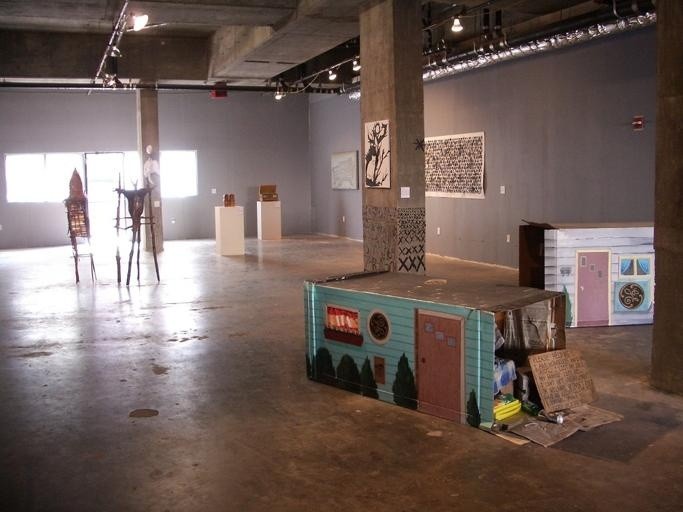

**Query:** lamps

left=351, top=57, right=360, bottom=73
left=450, top=17, right=463, bottom=34
left=131, top=13, right=148, bottom=32
left=326, top=68, right=336, bottom=81
